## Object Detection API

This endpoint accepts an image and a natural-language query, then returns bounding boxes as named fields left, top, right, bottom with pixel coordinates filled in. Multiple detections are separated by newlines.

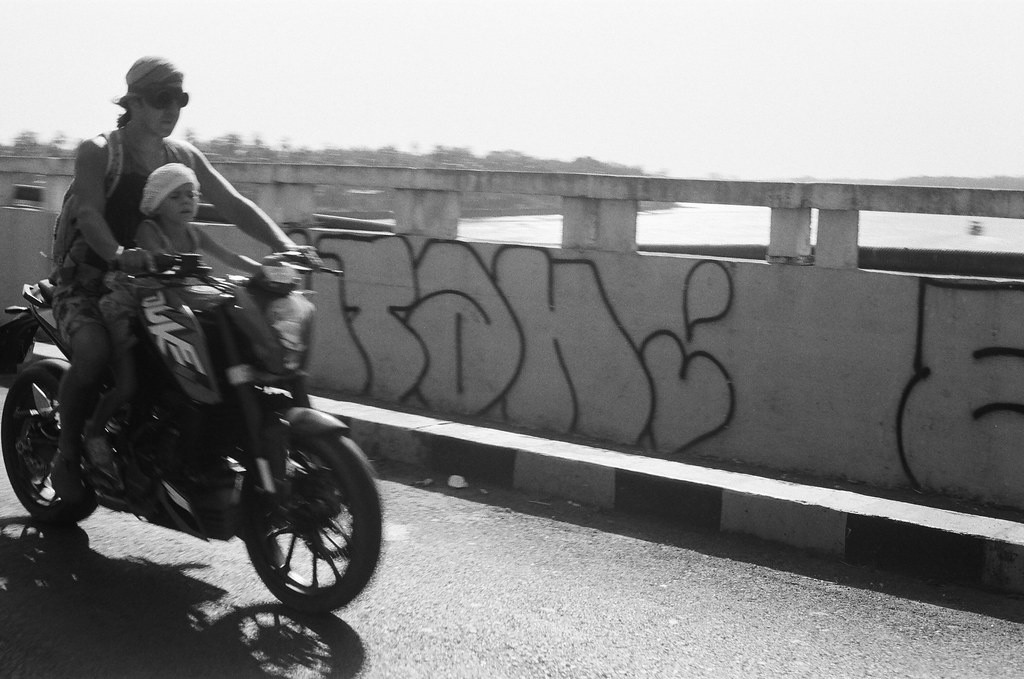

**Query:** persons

left=50, top=59, right=325, bottom=506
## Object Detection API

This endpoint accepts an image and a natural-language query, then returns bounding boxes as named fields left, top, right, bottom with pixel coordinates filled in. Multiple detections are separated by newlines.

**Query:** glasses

left=135, top=85, right=189, bottom=109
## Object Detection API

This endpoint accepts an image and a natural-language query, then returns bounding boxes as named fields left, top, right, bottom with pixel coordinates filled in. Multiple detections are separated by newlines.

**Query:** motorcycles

left=0, top=249, right=383, bottom=616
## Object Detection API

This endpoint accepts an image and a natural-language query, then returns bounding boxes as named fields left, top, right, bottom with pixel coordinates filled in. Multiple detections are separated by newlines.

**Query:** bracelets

left=115, top=245, right=125, bottom=256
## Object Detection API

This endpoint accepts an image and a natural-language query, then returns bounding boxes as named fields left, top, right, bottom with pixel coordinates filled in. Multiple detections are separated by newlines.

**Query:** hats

left=127, top=55, right=184, bottom=90
left=139, top=162, right=201, bottom=215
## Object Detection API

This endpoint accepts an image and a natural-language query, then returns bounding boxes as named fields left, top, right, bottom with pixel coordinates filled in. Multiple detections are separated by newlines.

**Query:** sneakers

left=83, top=428, right=113, bottom=467
left=49, top=448, right=80, bottom=500
